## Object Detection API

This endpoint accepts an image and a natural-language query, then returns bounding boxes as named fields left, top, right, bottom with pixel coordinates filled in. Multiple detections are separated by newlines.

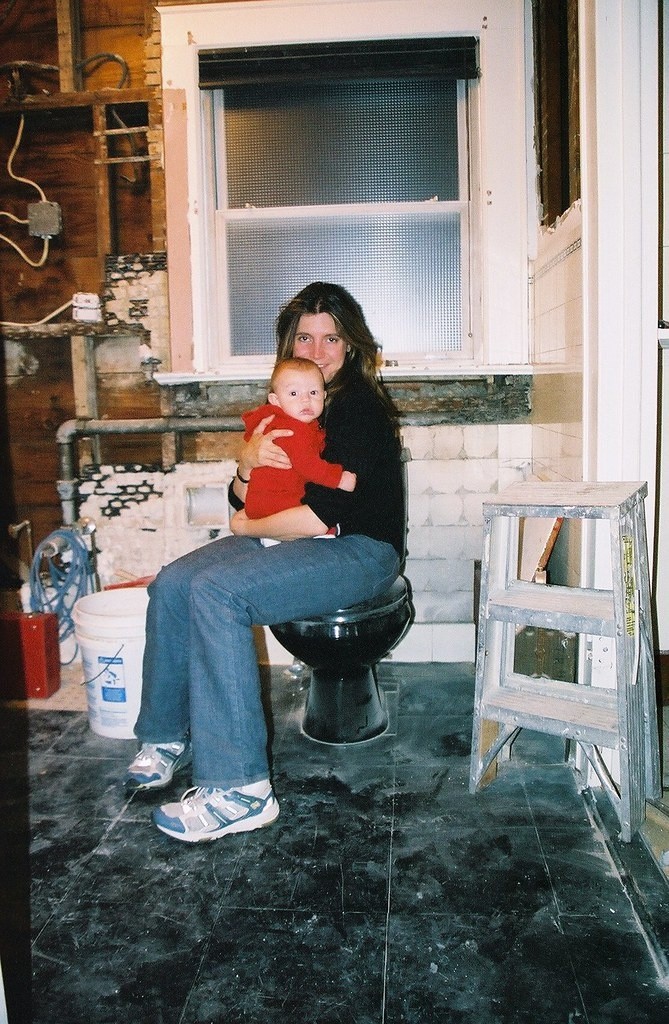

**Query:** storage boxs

left=0, top=613, right=61, bottom=700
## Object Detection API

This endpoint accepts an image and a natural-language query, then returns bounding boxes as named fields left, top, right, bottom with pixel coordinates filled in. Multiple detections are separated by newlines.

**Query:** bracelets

left=235, top=466, right=250, bottom=484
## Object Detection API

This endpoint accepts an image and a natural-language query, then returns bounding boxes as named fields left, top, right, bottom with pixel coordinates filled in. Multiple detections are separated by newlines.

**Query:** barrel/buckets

left=21, top=584, right=82, bottom=664
left=70, top=588, right=151, bottom=739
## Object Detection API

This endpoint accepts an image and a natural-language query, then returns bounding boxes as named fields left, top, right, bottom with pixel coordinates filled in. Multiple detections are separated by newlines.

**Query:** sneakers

left=125, top=741, right=193, bottom=793
left=153, top=779, right=280, bottom=843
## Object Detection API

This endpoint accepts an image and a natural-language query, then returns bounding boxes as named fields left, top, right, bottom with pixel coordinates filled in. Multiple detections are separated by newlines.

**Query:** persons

left=123, top=280, right=408, bottom=843
left=239, top=357, right=359, bottom=549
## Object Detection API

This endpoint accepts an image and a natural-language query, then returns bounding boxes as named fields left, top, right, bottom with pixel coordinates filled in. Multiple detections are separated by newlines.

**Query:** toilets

left=269, top=576, right=416, bottom=743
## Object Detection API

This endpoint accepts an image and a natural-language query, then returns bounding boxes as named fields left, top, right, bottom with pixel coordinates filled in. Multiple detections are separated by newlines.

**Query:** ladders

left=466, top=480, right=662, bottom=843
